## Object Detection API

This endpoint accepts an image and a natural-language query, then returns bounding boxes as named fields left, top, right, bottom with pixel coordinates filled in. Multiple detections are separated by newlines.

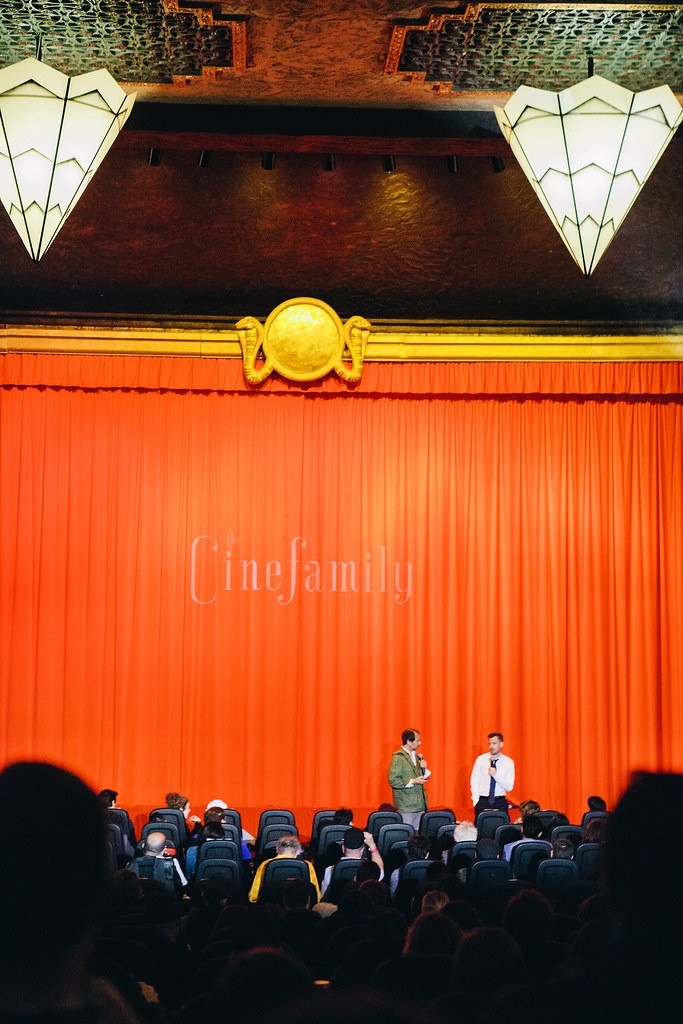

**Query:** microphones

left=489, top=757, right=494, bottom=769
left=419, top=753, right=426, bottom=775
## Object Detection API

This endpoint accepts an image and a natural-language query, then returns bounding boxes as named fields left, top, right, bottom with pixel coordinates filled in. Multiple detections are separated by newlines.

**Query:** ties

left=489, top=759, right=498, bottom=804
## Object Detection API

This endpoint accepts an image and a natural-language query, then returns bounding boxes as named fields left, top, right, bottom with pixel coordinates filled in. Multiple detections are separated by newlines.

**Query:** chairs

left=94, top=808, right=610, bottom=1024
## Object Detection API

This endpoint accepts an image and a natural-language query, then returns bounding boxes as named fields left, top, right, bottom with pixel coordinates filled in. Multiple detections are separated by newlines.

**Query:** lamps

left=0, top=35, right=137, bottom=263
left=493, top=51, right=683, bottom=281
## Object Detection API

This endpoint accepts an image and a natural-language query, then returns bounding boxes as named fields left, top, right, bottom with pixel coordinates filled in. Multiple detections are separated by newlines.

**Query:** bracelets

left=368, top=847, right=377, bottom=853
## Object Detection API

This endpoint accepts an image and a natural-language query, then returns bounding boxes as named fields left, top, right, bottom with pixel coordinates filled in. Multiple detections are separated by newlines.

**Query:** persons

left=503, top=815, right=544, bottom=861
left=0, top=758, right=123, bottom=1024
left=98, top=789, right=119, bottom=809
left=519, top=799, right=541, bottom=821
left=205, top=799, right=229, bottom=810
left=388, top=728, right=429, bottom=836
left=148, top=812, right=164, bottom=823
left=388, top=820, right=477, bottom=904
left=203, top=806, right=252, bottom=860
left=334, top=809, right=353, bottom=825
left=126, top=832, right=188, bottom=886
left=321, top=827, right=385, bottom=894
left=316, top=819, right=334, bottom=840
left=588, top=796, right=607, bottom=811
left=185, top=822, right=226, bottom=892
left=599, top=768, right=683, bottom=1024
left=118, top=871, right=603, bottom=1024
left=165, top=792, right=201, bottom=833
left=250, top=835, right=322, bottom=904
left=470, top=733, right=515, bottom=827
left=584, top=831, right=600, bottom=842
left=551, top=837, right=575, bottom=860
left=548, top=813, right=569, bottom=825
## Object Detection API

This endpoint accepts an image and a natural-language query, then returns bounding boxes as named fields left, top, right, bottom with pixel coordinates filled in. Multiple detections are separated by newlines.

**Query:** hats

left=345, top=828, right=363, bottom=849
left=205, top=799, right=228, bottom=811
